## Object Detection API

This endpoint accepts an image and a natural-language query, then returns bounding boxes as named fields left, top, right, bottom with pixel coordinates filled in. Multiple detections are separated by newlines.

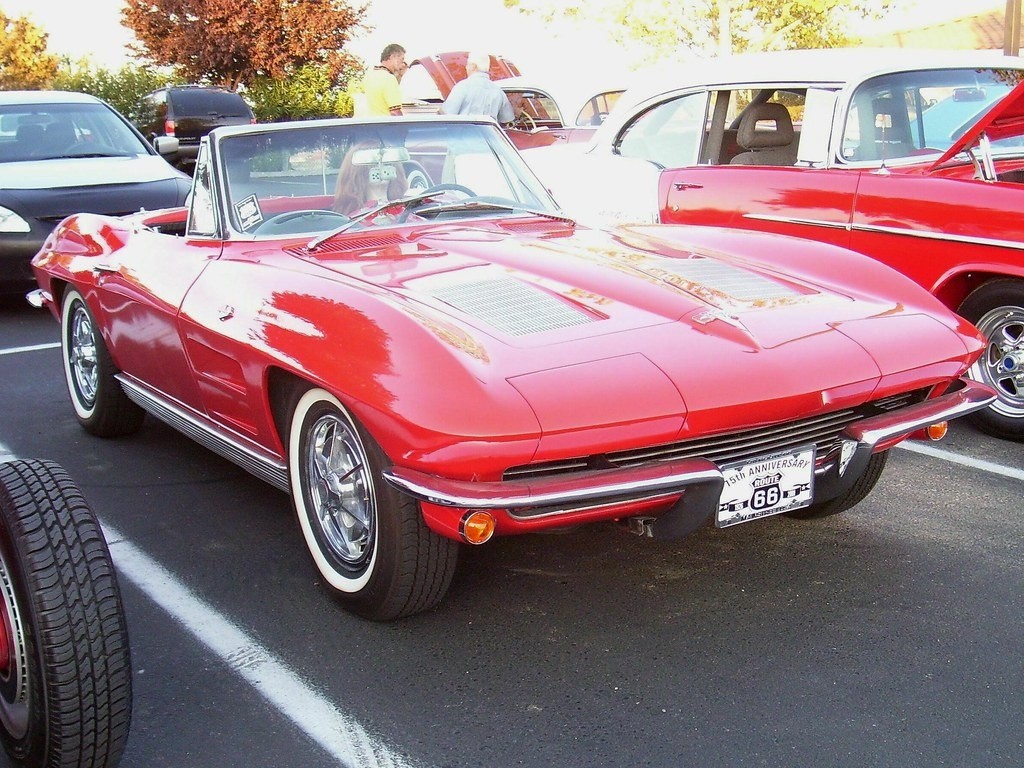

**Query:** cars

left=401, top=48, right=628, bottom=147
left=656, top=74, right=1024, bottom=440
left=910, top=84, right=1024, bottom=152
left=29, top=116, right=1000, bottom=623
left=584, top=49, right=1024, bottom=227
left=0, top=91, right=193, bottom=304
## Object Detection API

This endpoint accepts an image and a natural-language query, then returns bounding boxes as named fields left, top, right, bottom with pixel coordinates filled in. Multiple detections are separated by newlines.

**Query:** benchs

left=140, top=195, right=334, bottom=238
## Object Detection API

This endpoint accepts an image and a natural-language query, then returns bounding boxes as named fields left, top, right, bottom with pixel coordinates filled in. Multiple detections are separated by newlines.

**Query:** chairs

left=852, top=98, right=916, bottom=160
left=730, top=103, right=797, bottom=166
left=31, top=122, right=76, bottom=154
left=16, top=125, right=43, bottom=140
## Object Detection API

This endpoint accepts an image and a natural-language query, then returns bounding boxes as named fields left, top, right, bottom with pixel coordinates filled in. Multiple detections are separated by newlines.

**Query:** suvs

left=127, top=85, right=256, bottom=177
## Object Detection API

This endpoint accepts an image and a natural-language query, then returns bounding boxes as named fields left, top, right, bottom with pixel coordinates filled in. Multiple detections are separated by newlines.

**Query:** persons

left=365, top=44, right=407, bottom=146
left=437, top=51, right=514, bottom=184
left=331, top=139, right=428, bottom=227
